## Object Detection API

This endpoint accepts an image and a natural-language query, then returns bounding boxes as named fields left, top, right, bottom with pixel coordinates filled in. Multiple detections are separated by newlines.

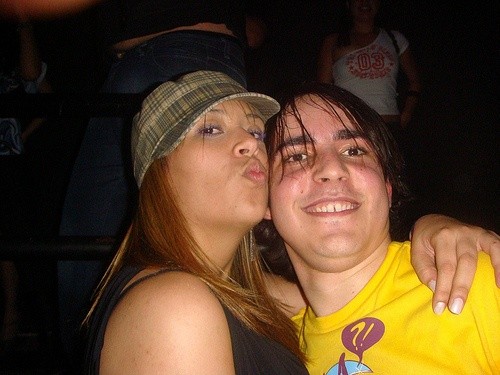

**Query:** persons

left=1, top=0, right=307, bottom=375
left=76, top=70, right=500, bottom=375
left=264, top=83, right=500, bottom=375
left=316, top=0, right=424, bottom=150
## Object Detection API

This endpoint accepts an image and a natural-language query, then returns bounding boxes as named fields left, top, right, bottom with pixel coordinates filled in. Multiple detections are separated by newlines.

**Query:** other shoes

left=2, top=311, right=19, bottom=341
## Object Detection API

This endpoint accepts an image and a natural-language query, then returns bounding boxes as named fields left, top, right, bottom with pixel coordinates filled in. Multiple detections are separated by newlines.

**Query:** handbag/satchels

left=384, top=27, right=417, bottom=113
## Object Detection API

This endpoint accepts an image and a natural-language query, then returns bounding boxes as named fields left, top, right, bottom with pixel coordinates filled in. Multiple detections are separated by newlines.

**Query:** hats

left=131, top=70, right=280, bottom=189
